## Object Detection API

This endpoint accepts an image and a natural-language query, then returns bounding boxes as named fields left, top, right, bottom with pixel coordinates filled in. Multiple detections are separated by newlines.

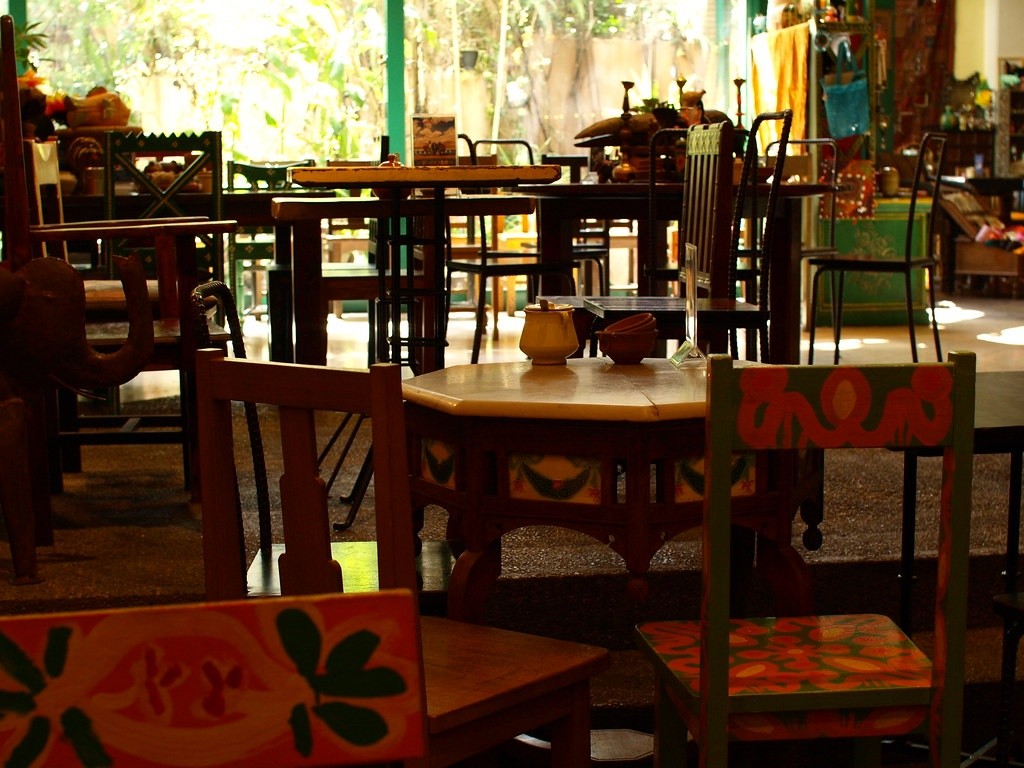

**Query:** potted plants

left=458, top=45, right=478, bottom=70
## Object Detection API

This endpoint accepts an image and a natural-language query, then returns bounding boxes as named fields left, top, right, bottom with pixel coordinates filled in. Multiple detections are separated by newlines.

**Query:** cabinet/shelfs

left=995, top=86, right=1023, bottom=180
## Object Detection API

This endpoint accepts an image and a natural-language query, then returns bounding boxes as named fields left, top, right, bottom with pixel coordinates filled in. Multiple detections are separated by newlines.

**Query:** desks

left=287, top=165, right=564, bottom=533
left=269, top=195, right=536, bottom=493
left=886, top=369, right=1023, bottom=768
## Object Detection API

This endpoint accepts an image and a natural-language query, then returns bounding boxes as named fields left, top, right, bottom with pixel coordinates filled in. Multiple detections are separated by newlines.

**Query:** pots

left=749, top=168, right=774, bottom=181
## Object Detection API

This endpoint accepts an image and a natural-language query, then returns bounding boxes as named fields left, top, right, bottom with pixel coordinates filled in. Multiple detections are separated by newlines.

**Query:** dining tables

left=512, top=181, right=850, bottom=365
left=398, top=356, right=814, bottom=726
left=28, top=189, right=335, bottom=366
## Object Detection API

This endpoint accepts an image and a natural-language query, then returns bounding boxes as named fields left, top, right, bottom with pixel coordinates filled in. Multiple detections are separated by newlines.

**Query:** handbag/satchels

left=819, top=41, right=870, bottom=139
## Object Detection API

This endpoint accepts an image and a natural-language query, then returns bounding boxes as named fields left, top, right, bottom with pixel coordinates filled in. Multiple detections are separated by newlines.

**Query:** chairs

left=23, top=107, right=837, bottom=364
left=190, top=279, right=448, bottom=615
left=2, top=216, right=237, bottom=494
left=0, top=588, right=427, bottom=768
left=808, top=132, right=948, bottom=363
left=192, top=347, right=612, bottom=768
left=635, top=348, right=976, bottom=767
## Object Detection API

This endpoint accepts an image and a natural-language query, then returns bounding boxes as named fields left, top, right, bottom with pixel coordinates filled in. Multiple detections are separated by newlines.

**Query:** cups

left=519, top=302, right=579, bottom=365
left=82, top=166, right=104, bottom=194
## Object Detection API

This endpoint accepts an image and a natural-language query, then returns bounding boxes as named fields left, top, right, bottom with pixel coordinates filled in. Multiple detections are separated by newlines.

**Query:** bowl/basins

left=372, top=155, right=412, bottom=199
left=596, top=313, right=659, bottom=363
